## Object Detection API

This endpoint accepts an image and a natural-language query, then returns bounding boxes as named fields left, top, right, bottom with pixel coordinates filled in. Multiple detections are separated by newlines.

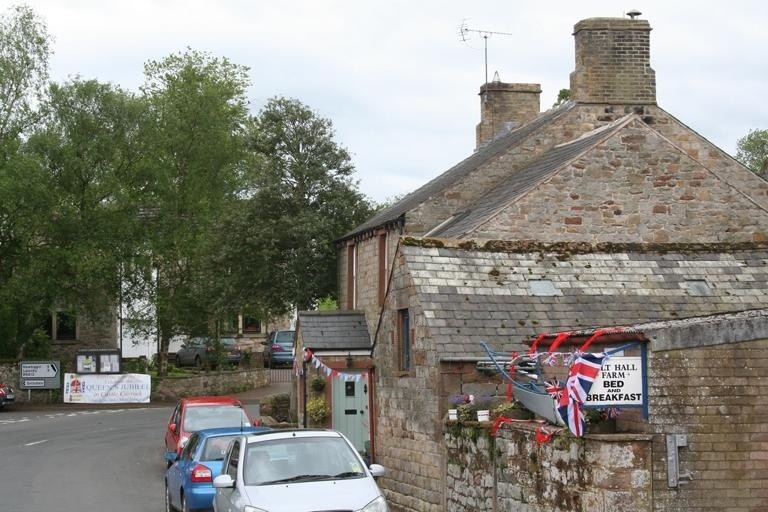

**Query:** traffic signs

left=19, top=361, right=59, bottom=388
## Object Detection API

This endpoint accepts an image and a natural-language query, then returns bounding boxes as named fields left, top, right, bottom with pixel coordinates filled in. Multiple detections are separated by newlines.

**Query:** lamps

left=345, top=350, right=354, bottom=369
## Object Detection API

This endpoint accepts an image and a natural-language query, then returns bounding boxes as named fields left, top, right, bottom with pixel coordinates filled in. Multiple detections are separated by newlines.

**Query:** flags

left=544, top=352, right=621, bottom=439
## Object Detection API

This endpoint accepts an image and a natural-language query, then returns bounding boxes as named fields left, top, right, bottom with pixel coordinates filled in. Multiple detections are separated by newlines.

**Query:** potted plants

left=445, top=391, right=498, bottom=423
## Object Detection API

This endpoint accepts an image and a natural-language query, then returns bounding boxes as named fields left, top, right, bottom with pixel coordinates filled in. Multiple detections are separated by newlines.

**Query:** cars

left=213, top=430, right=389, bottom=512
left=257, top=326, right=297, bottom=367
left=161, top=426, right=278, bottom=512
left=162, top=394, right=263, bottom=454
left=173, top=334, right=242, bottom=369
